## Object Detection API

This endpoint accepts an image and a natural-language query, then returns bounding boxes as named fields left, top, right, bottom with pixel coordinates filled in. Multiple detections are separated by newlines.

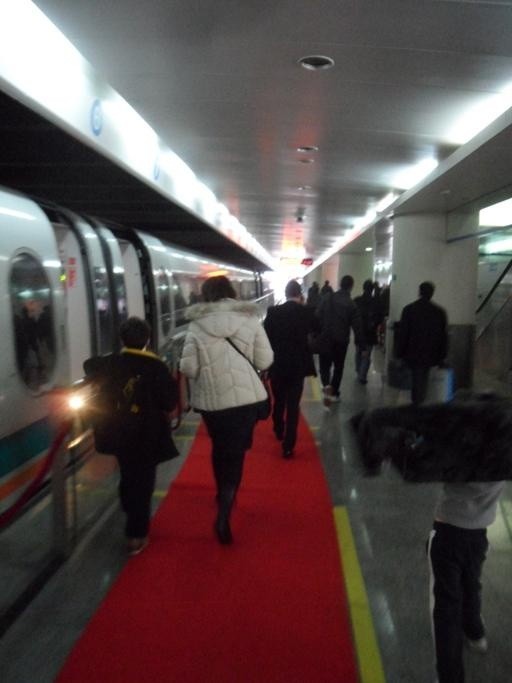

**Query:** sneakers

left=466, top=615, right=490, bottom=653
left=125, top=536, right=148, bottom=555
left=320, top=386, right=342, bottom=402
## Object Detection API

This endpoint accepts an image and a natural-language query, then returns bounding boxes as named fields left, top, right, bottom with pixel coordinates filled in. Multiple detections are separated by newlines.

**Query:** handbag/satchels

left=429, top=367, right=454, bottom=405
left=377, top=324, right=385, bottom=345
left=261, top=379, right=272, bottom=419
left=388, top=359, right=413, bottom=391
left=94, top=417, right=123, bottom=454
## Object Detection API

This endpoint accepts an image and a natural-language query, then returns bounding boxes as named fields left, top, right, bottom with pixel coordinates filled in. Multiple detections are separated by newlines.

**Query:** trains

left=0, top=188, right=274, bottom=639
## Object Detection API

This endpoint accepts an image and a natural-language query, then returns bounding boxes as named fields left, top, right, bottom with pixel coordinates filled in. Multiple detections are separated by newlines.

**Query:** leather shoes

left=273, top=425, right=292, bottom=458
left=213, top=517, right=233, bottom=545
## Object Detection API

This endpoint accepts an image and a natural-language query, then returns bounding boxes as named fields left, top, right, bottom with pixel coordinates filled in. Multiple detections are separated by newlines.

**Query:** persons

left=82, top=318, right=179, bottom=553
left=349, top=387, right=511, bottom=682
left=180, top=274, right=273, bottom=543
left=395, top=281, right=450, bottom=402
left=307, top=275, right=390, bottom=414
left=264, top=280, right=320, bottom=459
left=11, top=304, right=51, bottom=387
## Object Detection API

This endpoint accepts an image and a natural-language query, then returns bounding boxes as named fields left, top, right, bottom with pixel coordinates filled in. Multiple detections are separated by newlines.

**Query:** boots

left=355, top=368, right=368, bottom=385
